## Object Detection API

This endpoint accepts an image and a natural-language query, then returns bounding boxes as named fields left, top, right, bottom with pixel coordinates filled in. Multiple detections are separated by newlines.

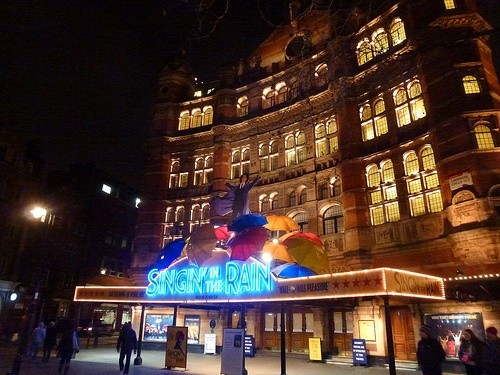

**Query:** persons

left=223, top=173, right=261, bottom=218
left=27, top=322, right=46, bottom=362
left=41, top=322, right=57, bottom=362
left=55, top=324, right=80, bottom=375
left=116, top=322, right=137, bottom=375
left=416, top=325, right=445, bottom=375
left=481, top=327, right=500, bottom=375
left=458, top=329, right=483, bottom=375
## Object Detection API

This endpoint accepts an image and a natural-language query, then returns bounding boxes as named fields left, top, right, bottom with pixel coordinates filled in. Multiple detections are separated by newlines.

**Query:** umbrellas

left=152, top=214, right=328, bottom=279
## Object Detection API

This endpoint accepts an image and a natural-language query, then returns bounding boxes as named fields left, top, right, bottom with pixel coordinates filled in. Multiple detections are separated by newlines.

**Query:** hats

left=420, top=325, right=431, bottom=334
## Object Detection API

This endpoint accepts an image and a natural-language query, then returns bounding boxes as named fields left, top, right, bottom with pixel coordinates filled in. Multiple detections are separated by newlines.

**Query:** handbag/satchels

left=72, top=331, right=80, bottom=350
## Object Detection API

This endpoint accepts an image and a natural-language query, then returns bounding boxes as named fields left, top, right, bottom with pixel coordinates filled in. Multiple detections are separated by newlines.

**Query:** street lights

left=8, top=201, right=59, bottom=373
